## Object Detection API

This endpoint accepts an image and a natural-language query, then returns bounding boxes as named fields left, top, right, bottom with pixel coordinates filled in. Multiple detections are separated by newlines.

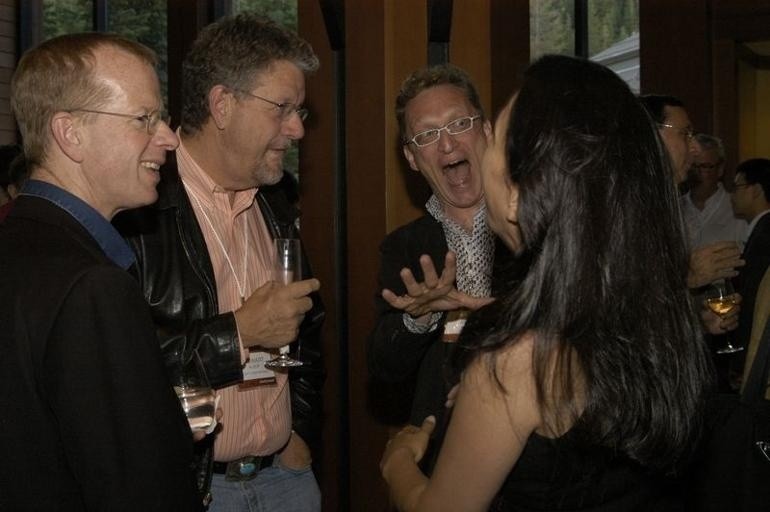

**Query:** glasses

left=406, top=115, right=484, bottom=149
left=693, top=161, right=722, bottom=173
left=656, top=121, right=696, bottom=141
left=59, top=107, right=172, bottom=136
left=234, top=87, right=310, bottom=123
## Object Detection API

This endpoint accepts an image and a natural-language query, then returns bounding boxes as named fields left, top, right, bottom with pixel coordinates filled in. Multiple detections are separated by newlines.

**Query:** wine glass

left=264, top=238, right=305, bottom=368
left=707, top=281, right=743, bottom=354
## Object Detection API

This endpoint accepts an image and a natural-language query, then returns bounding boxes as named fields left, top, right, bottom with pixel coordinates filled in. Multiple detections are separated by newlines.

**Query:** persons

left=379, top=55, right=714, bottom=510
left=640, top=95, right=769, bottom=511
left=369, top=65, right=491, bottom=478
left=2, top=33, right=217, bottom=512
left=109, top=11, right=321, bottom=512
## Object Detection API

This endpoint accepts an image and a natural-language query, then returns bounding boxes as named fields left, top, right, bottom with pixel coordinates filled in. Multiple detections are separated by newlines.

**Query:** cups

left=172, top=383, right=215, bottom=434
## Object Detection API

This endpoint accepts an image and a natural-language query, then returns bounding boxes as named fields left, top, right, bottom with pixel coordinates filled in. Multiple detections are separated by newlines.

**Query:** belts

left=213, top=435, right=294, bottom=483
